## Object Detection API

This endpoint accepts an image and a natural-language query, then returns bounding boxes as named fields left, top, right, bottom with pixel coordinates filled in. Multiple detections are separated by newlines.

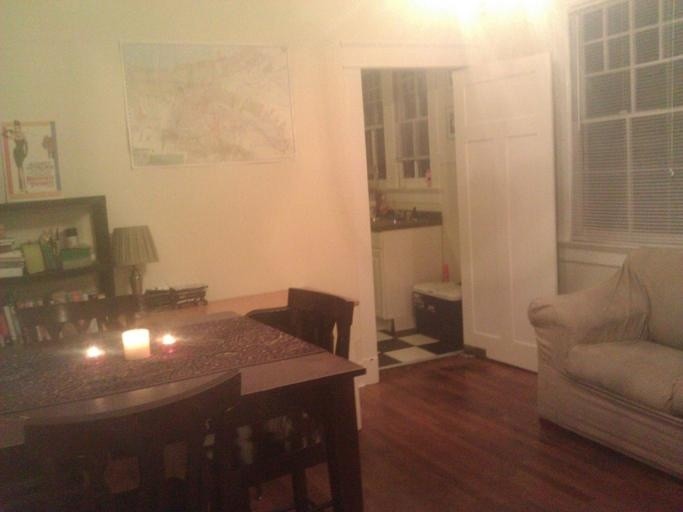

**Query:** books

left=0, top=120, right=61, bottom=203
left=0, top=236, right=106, bottom=346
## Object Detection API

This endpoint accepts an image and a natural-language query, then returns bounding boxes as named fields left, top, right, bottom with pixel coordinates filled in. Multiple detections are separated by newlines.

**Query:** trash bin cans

left=413, top=284, right=462, bottom=346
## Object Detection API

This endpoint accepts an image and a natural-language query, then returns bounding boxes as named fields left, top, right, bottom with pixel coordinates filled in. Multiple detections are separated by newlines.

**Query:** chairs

left=526, top=245, right=683, bottom=481
left=23, top=367, right=240, bottom=512
left=15, top=294, right=142, bottom=343
left=188, top=287, right=354, bottom=512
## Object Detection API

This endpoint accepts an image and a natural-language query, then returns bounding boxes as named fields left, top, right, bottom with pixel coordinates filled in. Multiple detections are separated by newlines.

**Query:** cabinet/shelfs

left=371, top=225, right=444, bottom=334
left=0, top=194, right=116, bottom=346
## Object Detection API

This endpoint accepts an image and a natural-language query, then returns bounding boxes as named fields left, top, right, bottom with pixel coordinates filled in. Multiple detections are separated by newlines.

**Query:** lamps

left=111, top=223, right=159, bottom=311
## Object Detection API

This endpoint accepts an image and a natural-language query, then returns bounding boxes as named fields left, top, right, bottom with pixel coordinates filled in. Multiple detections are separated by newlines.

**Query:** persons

left=3, top=120, right=32, bottom=193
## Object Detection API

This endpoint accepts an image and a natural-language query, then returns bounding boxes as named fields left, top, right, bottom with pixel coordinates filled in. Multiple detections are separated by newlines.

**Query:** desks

left=0, top=310, right=366, bottom=512
